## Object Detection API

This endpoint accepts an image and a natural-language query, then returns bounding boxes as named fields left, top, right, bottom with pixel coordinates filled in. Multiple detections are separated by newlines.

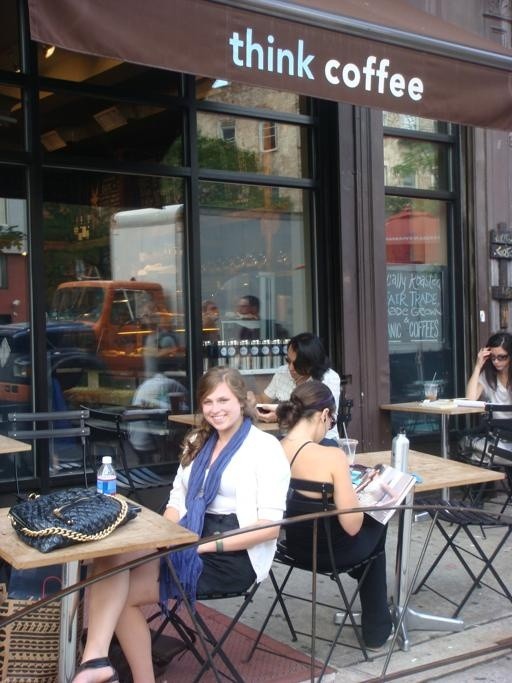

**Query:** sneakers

left=366, top=623, right=394, bottom=652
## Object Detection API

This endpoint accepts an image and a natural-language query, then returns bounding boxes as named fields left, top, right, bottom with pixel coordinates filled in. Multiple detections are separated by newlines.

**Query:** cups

left=168, top=393, right=185, bottom=413
left=424, top=384, right=439, bottom=401
left=336, top=438, right=358, bottom=466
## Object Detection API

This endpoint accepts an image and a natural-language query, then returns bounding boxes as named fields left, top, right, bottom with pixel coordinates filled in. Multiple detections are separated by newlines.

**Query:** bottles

left=73, top=215, right=93, bottom=241
left=391, top=427, right=410, bottom=473
left=96, top=456, right=116, bottom=497
left=202, top=339, right=289, bottom=369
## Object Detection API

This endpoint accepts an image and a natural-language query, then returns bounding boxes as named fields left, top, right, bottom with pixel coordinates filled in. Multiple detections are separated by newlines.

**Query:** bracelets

left=214, top=530, right=224, bottom=554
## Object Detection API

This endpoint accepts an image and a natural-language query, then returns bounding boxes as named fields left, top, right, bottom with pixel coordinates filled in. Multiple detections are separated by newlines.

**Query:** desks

left=2, top=489, right=198, bottom=681
left=343, top=447, right=507, bottom=655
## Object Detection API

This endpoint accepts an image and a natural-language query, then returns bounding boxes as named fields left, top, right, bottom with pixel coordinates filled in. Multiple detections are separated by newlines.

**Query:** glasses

left=286, top=359, right=295, bottom=365
left=330, top=414, right=335, bottom=430
left=490, top=354, right=509, bottom=361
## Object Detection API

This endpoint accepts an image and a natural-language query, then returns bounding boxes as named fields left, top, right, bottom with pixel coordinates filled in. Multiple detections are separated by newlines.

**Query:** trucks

left=0, top=203, right=304, bottom=441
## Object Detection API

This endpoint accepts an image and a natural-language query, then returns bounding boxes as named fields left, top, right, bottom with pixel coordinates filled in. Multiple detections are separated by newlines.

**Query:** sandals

left=70, top=658, right=119, bottom=683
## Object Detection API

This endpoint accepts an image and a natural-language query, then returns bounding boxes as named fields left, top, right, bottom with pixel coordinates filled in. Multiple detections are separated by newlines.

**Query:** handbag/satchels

left=8, top=487, right=141, bottom=553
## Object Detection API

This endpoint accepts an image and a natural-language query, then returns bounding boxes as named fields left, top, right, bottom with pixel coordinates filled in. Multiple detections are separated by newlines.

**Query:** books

left=350, top=463, right=417, bottom=526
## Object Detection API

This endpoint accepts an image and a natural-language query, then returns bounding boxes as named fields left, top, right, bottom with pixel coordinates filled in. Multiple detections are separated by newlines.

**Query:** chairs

left=2, top=397, right=511, bottom=513
left=408, top=426, right=510, bottom=620
left=149, top=489, right=299, bottom=681
left=238, top=478, right=384, bottom=681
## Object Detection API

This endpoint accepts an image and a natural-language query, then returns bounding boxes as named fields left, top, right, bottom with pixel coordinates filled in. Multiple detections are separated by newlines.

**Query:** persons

left=355, top=464, right=399, bottom=515
left=129, top=368, right=190, bottom=463
left=245, top=332, right=342, bottom=447
left=140, top=299, right=186, bottom=376
left=238, top=296, right=288, bottom=340
left=277, top=381, right=397, bottom=652
left=202, top=299, right=222, bottom=342
left=71, top=366, right=294, bottom=682
left=458, top=332, right=512, bottom=498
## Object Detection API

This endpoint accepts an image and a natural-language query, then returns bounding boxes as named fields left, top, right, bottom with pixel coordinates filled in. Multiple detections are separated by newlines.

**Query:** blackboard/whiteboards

left=386, top=262, right=450, bottom=355
